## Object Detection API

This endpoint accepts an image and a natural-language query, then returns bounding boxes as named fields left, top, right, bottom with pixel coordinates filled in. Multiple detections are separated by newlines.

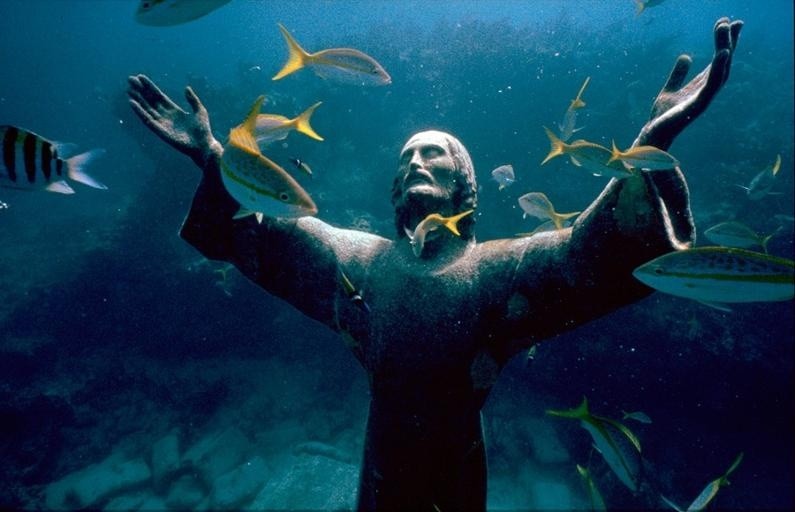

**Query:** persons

left=123, top=16, right=747, bottom=512
